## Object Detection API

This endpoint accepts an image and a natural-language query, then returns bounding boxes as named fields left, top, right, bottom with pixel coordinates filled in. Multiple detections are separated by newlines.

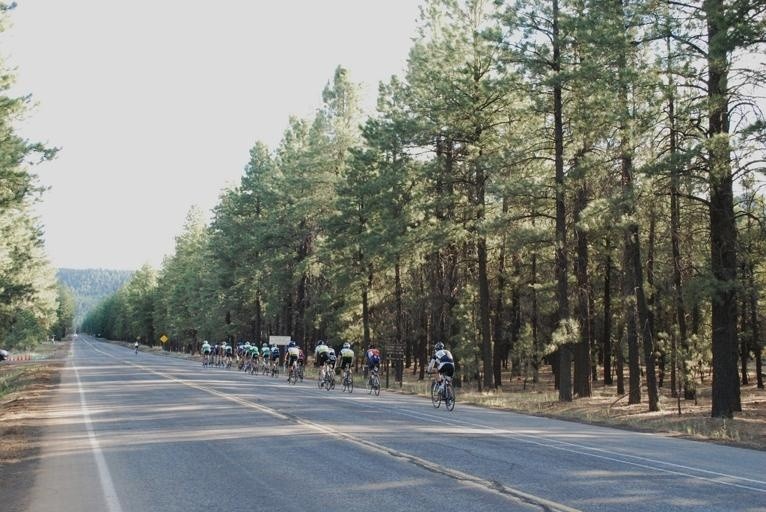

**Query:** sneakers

left=320, top=379, right=350, bottom=386
left=203, top=362, right=278, bottom=374
left=368, top=381, right=378, bottom=389
left=438, top=390, right=453, bottom=402
left=287, top=375, right=304, bottom=382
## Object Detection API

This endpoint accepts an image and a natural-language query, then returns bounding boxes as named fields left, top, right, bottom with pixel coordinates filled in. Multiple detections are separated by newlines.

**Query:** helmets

left=317, top=340, right=324, bottom=343
left=288, top=342, right=295, bottom=345
left=368, top=343, right=374, bottom=347
left=435, top=342, right=444, bottom=350
left=204, top=340, right=277, bottom=346
left=343, top=343, right=350, bottom=347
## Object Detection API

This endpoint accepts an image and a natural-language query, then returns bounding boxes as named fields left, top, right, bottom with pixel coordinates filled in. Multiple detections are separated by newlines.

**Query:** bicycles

left=424, top=368, right=455, bottom=411
left=311, top=358, right=381, bottom=396
left=199, top=349, right=280, bottom=377
left=283, top=359, right=305, bottom=384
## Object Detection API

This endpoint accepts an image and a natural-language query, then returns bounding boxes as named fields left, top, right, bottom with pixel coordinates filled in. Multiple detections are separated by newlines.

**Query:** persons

left=200, top=340, right=280, bottom=377
left=134, top=340, right=138, bottom=352
left=337, top=342, right=354, bottom=384
left=426, top=341, right=455, bottom=401
left=328, top=346, right=336, bottom=370
left=296, top=344, right=304, bottom=371
left=312, top=340, right=329, bottom=388
left=284, top=340, right=299, bottom=375
left=362, top=343, right=381, bottom=382
